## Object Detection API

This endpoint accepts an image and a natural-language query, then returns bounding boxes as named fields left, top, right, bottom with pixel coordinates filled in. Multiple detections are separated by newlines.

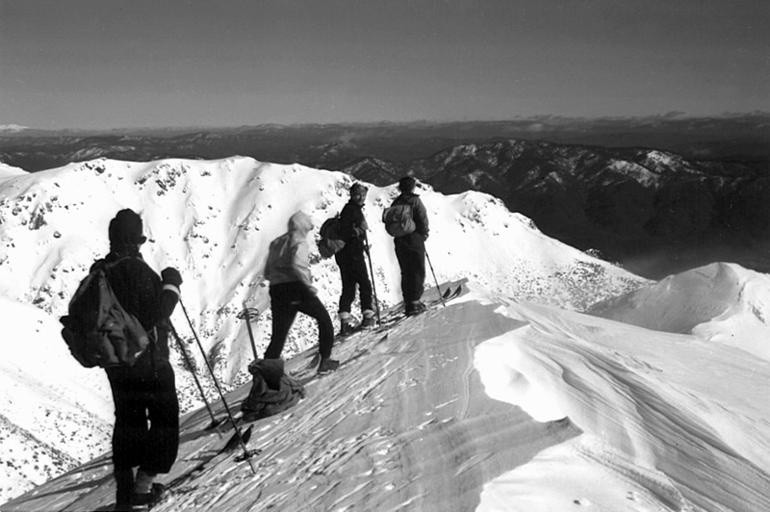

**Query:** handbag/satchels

left=241, top=359, right=306, bottom=422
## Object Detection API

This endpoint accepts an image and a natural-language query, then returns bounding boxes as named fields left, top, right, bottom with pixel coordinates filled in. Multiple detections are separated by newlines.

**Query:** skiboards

left=164, top=423, right=254, bottom=492
left=287, top=333, right=388, bottom=388
left=305, top=285, right=461, bottom=359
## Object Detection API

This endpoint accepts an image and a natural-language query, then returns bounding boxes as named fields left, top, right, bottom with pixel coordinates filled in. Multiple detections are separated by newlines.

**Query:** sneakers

left=341, top=317, right=359, bottom=335
left=134, top=483, right=165, bottom=504
left=363, top=313, right=377, bottom=327
left=318, top=358, right=339, bottom=374
left=405, top=301, right=425, bottom=316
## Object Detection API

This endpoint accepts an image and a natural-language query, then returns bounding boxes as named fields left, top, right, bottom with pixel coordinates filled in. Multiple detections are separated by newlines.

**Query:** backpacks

left=317, top=204, right=359, bottom=260
left=385, top=194, right=416, bottom=238
left=60, top=255, right=158, bottom=369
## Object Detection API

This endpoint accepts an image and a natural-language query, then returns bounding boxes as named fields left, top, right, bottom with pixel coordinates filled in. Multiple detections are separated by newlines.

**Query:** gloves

left=161, top=268, right=182, bottom=286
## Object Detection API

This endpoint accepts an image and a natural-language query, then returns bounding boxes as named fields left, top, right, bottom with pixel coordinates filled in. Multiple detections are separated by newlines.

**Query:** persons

left=392, top=175, right=431, bottom=318
left=103, top=207, right=183, bottom=512
left=335, top=182, right=384, bottom=336
left=263, top=209, right=340, bottom=376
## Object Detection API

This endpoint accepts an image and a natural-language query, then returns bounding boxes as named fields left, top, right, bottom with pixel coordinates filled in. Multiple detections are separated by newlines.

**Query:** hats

left=108, top=209, right=145, bottom=248
